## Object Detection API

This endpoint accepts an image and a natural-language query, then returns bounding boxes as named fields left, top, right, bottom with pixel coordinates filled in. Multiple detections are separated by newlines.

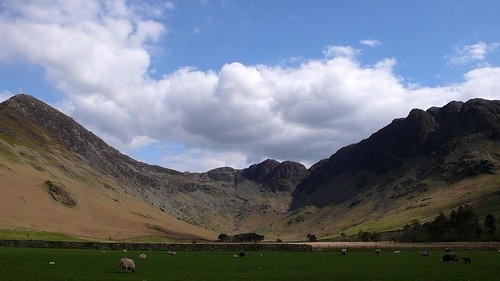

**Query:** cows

left=441, top=253, right=457, bottom=262
left=462, top=257, right=472, bottom=264
left=239, top=252, right=247, bottom=260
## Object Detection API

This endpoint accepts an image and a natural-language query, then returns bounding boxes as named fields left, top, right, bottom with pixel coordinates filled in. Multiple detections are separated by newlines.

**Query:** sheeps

left=233, top=254, right=238, bottom=258
left=168, top=251, right=176, bottom=255
left=394, top=251, right=401, bottom=254
left=119, top=258, right=135, bottom=272
left=340, top=249, right=347, bottom=254
left=49, top=262, right=56, bottom=265
left=417, top=251, right=432, bottom=256
left=139, top=253, right=146, bottom=260
left=376, top=249, right=382, bottom=253
